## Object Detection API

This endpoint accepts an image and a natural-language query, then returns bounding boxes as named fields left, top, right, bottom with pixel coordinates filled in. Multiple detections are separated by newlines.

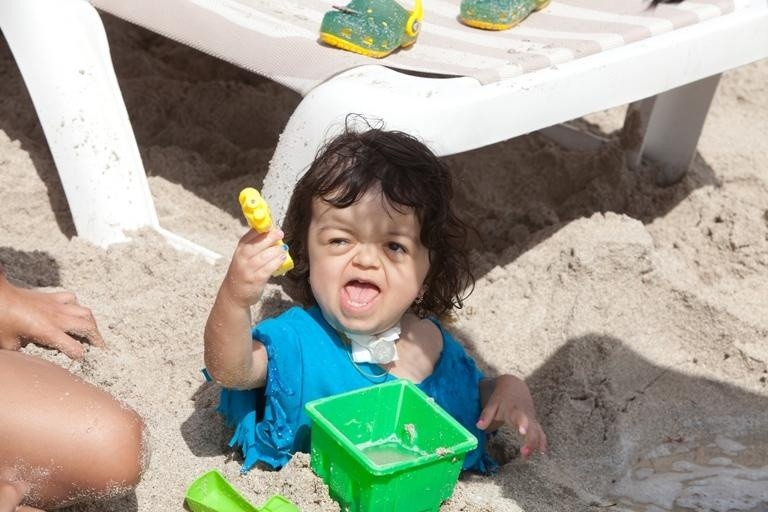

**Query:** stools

left=90, top=4, right=767, bottom=265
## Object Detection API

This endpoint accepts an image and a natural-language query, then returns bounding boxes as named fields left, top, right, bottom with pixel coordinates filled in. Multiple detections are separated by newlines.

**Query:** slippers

left=459, top=0, right=552, bottom=32
left=318, top=0, right=425, bottom=59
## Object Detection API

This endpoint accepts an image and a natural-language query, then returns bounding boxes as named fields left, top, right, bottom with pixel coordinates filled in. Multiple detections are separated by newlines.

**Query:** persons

left=1, top=268, right=148, bottom=512
left=205, top=113, right=546, bottom=473
